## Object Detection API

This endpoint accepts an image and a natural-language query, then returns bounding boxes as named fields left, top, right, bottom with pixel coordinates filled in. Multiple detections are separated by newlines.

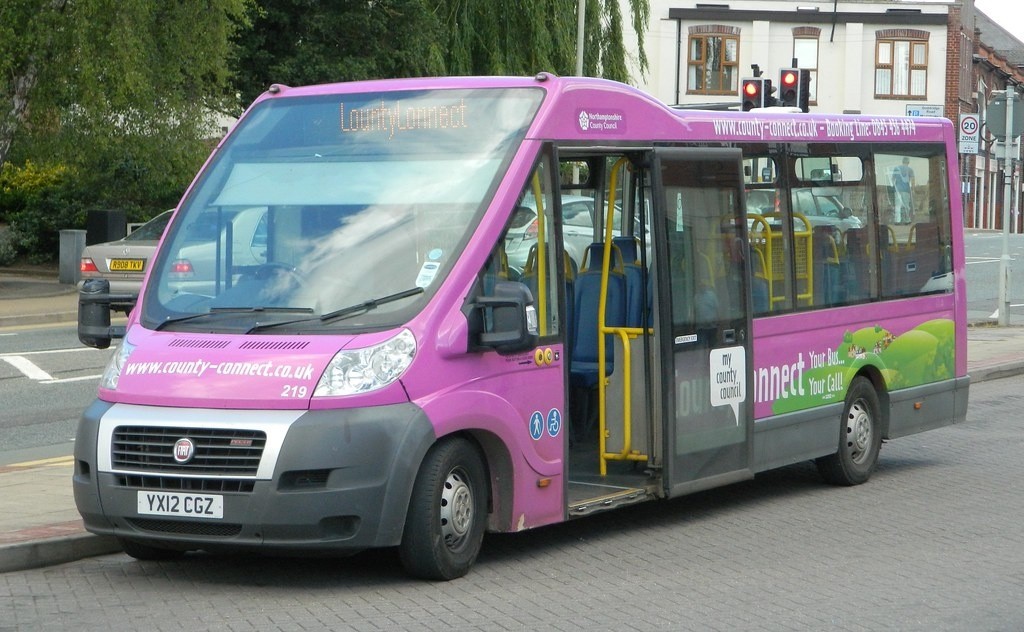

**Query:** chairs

left=477, top=212, right=957, bottom=434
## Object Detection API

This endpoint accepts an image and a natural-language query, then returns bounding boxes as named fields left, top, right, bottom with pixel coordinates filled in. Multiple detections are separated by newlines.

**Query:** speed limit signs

left=962, top=117, right=978, bottom=135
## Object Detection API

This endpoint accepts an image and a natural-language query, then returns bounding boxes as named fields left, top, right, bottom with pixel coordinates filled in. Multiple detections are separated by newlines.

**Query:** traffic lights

left=740, top=77, right=763, bottom=112
left=781, top=69, right=801, bottom=111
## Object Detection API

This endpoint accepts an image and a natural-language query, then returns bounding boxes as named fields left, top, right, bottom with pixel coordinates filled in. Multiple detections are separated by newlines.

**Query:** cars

left=78, top=208, right=268, bottom=321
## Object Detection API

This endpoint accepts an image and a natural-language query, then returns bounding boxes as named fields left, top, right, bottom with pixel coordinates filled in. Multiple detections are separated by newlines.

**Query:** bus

left=71, top=76, right=973, bottom=581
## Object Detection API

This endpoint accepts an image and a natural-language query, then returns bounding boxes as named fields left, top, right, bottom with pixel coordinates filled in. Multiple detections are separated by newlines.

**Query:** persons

left=892, top=157, right=915, bottom=225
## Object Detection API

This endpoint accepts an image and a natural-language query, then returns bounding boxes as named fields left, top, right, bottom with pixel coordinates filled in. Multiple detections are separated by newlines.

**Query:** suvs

left=731, top=181, right=863, bottom=241
left=503, top=194, right=652, bottom=279
